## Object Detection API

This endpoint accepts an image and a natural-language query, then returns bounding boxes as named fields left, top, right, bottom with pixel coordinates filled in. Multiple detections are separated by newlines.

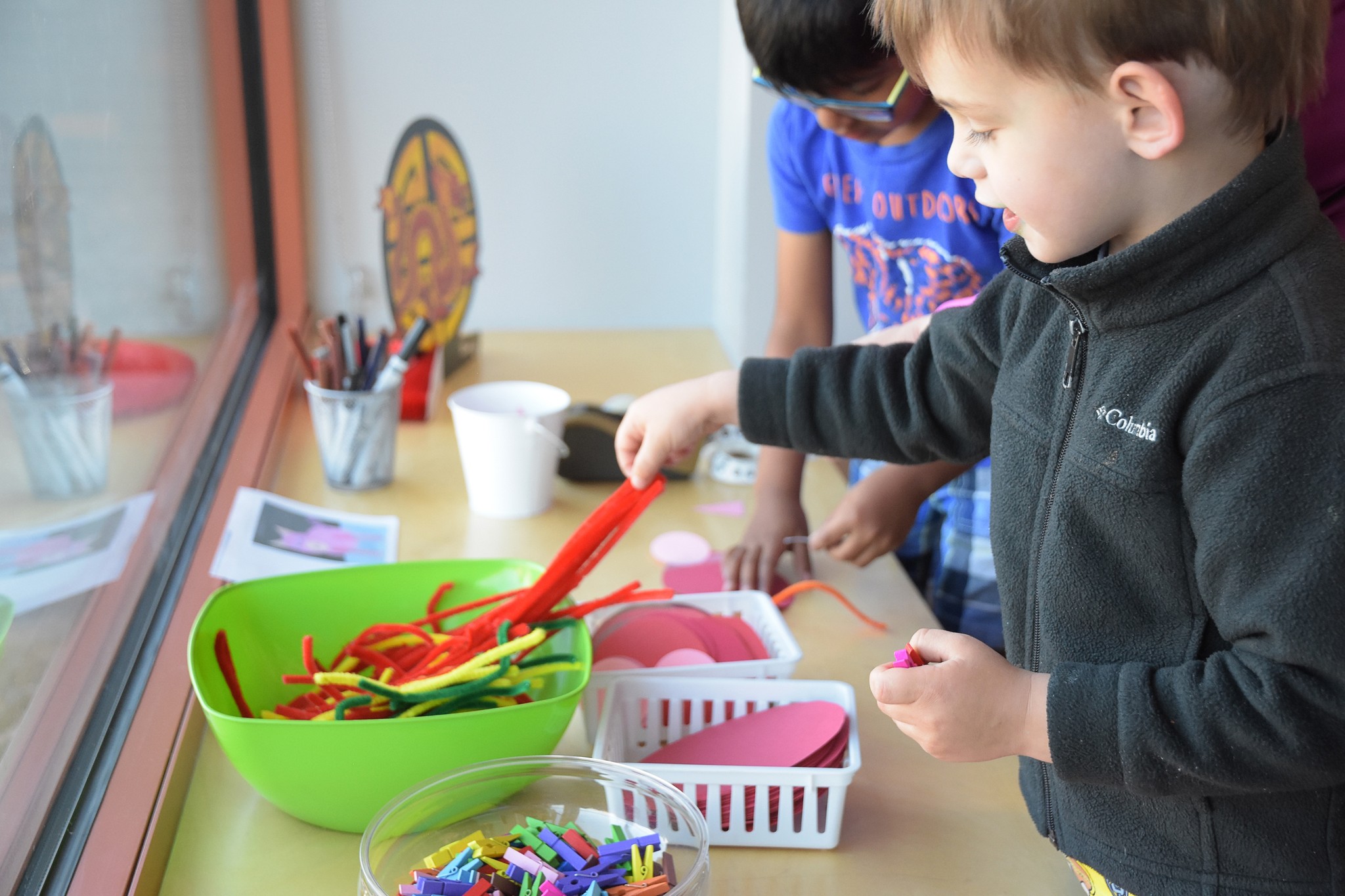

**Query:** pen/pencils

left=2, top=317, right=121, bottom=495
left=288, top=315, right=430, bottom=488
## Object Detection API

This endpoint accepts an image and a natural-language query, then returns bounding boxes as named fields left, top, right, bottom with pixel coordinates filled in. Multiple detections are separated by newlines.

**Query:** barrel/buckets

left=447, top=380, right=572, bottom=517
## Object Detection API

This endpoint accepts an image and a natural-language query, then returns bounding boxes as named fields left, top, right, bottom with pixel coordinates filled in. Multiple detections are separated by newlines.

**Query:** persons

left=612, top=0, right=1345, bottom=896
left=726, top=0, right=1019, bottom=671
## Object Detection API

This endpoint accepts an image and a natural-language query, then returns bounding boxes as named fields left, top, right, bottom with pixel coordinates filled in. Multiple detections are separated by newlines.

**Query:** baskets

left=593, top=674, right=863, bottom=850
left=572, top=588, right=803, bottom=759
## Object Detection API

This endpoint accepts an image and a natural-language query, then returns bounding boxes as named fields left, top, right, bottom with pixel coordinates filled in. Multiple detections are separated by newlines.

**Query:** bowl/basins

left=354, top=753, right=711, bottom=896
left=186, top=556, right=594, bottom=837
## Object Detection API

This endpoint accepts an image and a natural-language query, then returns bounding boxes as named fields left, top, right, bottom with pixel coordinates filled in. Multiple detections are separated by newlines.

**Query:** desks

left=0, top=335, right=219, bottom=759
left=157, top=323, right=1089, bottom=896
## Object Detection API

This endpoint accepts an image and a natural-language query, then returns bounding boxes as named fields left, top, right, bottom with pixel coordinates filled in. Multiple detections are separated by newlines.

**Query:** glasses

left=750, top=65, right=909, bottom=125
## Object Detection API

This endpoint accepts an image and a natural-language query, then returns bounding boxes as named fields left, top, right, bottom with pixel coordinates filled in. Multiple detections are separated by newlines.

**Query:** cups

left=0, top=373, right=114, bottom=504
left=305, top=371, right=405, bottom=492
